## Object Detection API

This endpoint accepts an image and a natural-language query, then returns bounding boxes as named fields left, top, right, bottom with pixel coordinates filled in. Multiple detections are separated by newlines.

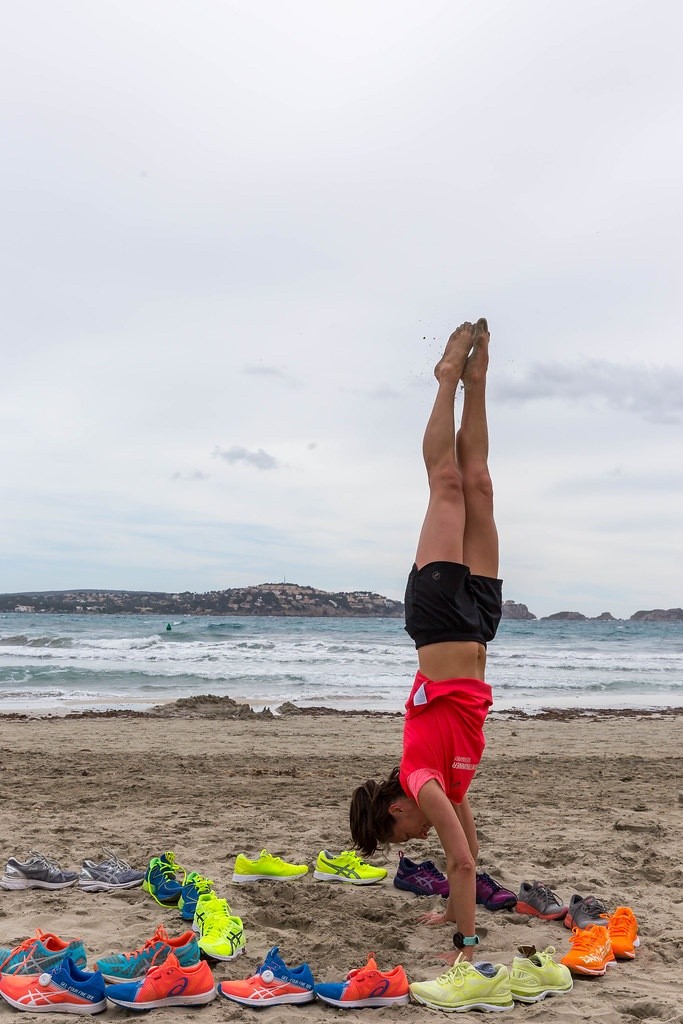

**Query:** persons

left=348, top=317, right=503, bottom=965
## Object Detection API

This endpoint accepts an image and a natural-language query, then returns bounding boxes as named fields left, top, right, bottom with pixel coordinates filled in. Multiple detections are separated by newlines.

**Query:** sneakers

left=191, top=891, right=233, bottom=938
left=395, top=851, right=451, bottom=897
left=564, top=894, right=610, bottom=931
left=198, top=916, right=247, bottom=961
left=0, top=952, right=107, bottom=1014
left=516, top=880, right=569, bottom=919
left=560, top=924, right=618, bottom=975
left=232, top=850, right=309, bottom=882
left=511, top=946, right=573, bottom=1003
left=2, top=852, right=79, bottom=890
left=599, top=906, right=640, bottom=959
left=312, top=952, right=408, bottom=1007
left=94, top=924, right=201, bottom=984
left=475, top=873, right=519, bottom=910
left=177, top=872, right=213, bottom=919
left=0, top=928, right=88, bottom=971
left=141, top=852, right=187, bottom=908
left=217, top=948, right=315, bottom=1006
left=79, top=846, right=144, bottom=892
left=313, top=850, right=388, bottom=885
left=408, top=952, right=515, bottom=1013
left=103, top=954, right=216, bottom=1008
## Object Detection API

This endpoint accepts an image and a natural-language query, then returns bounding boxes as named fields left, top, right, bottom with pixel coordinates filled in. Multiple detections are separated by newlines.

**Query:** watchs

left=453, top=933, right=479, bottom=947
